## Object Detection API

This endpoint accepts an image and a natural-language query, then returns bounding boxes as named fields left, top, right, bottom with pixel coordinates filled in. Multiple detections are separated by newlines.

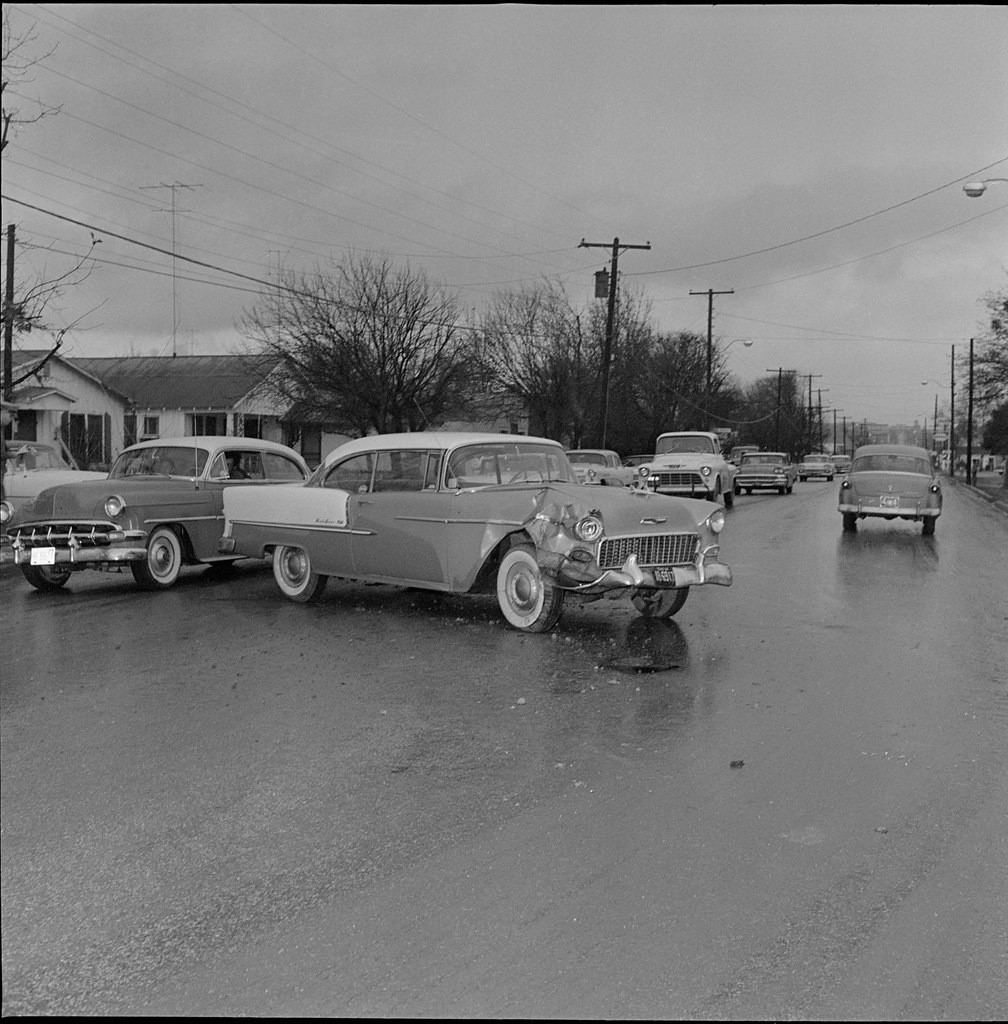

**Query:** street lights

left=921, top=377, right=954, bottom=477
left=703, top=338, right=754, bottom=429
left=817, top=398, right=834, bottom=453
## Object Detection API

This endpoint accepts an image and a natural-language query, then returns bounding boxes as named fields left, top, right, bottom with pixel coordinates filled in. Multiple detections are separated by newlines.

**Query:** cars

left=638, top=431, right=739, bottom=507
left=216, top=430, right=733, bottom=633
left=456, top=453, right=565, bottom=485
left=728, top=445, right=762, bottom=466
left=0, top=440, right=109, bottom=514
left=837, top=444, right=943, bottom=537
left=734, top=452, right=798, bottom=496
left=796, top=454, right=836, bottom=482
left=830, top=454, right=852, bottom=475
left=1, top=435, right=311, bottom=593
left=564, top=449, right=639, bottom=486
left=622, top=453, right=655, bottom=467
